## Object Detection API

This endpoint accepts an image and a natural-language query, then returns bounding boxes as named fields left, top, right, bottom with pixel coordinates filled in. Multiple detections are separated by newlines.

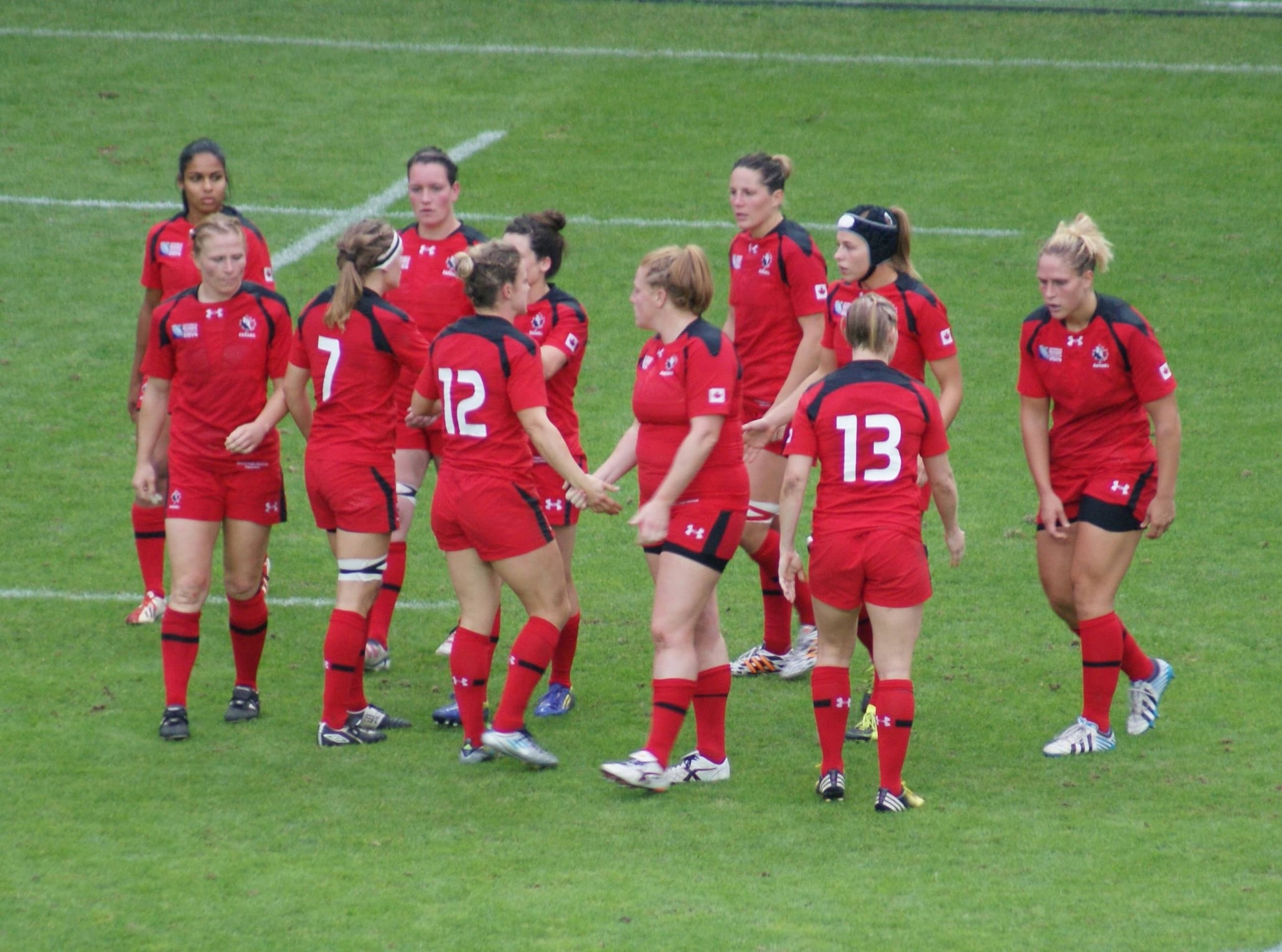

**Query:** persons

left=1015, top=207, right=1182, bottom=761
left=354, top=143, right=487, bottom=673
left=712, top=152, right=832, bottom=683
left=411, top=235, right=626, bottom=770
left=405, top=208, right=592, bottom=728
left=286, top=225, right=429, bottom=748
left=131, top=207, right=297, bottom=745
left=120, top=136, right=281, bottom=625
left=771, top=285, right=967, bottom=813
left=558, top=235, right=753, bottom=793
left=738, top=203, right=964, bottom=739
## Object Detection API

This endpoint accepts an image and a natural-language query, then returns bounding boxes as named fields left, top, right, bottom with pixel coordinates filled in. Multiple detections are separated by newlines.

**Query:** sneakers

left=317, top=718, right=388, bottom=748
left=533, top=683, right=574, bottom=717
left=432, top=694, right=490, bottom=727
left=260, top=552, right=270, bottom=594
left=778, top=624, right=818, bottom=678
left=1126, top=656, right=1177, bottom=736
left=435, top=629, right=456, bottom=659
left=668, top=750, right=731, bottom=784
left=479, top=723, right=559, bottom=765
left=224, top=683, right=260, bottom=722
left=460, top=738, right=506, bottom=763
left=874, top=781, right=924, bottom=812
left=158, top=706, right=189, bottom=740
left=363, top=639, right=391, bottom=672
left=1043, top=715, right=1116, bottom=757
left=599, top=750, right=671, bottom=792
left=814, top=768, right=845, bottom=802
left=126, top=590, right=165, bottom=625
left=345, top=704, right=414, bottom=730
left=861, top=659, right=875, bottom=713
left=845, top=704, right=877, bottom=741
left=729, top=640, right=795, bottom=676
